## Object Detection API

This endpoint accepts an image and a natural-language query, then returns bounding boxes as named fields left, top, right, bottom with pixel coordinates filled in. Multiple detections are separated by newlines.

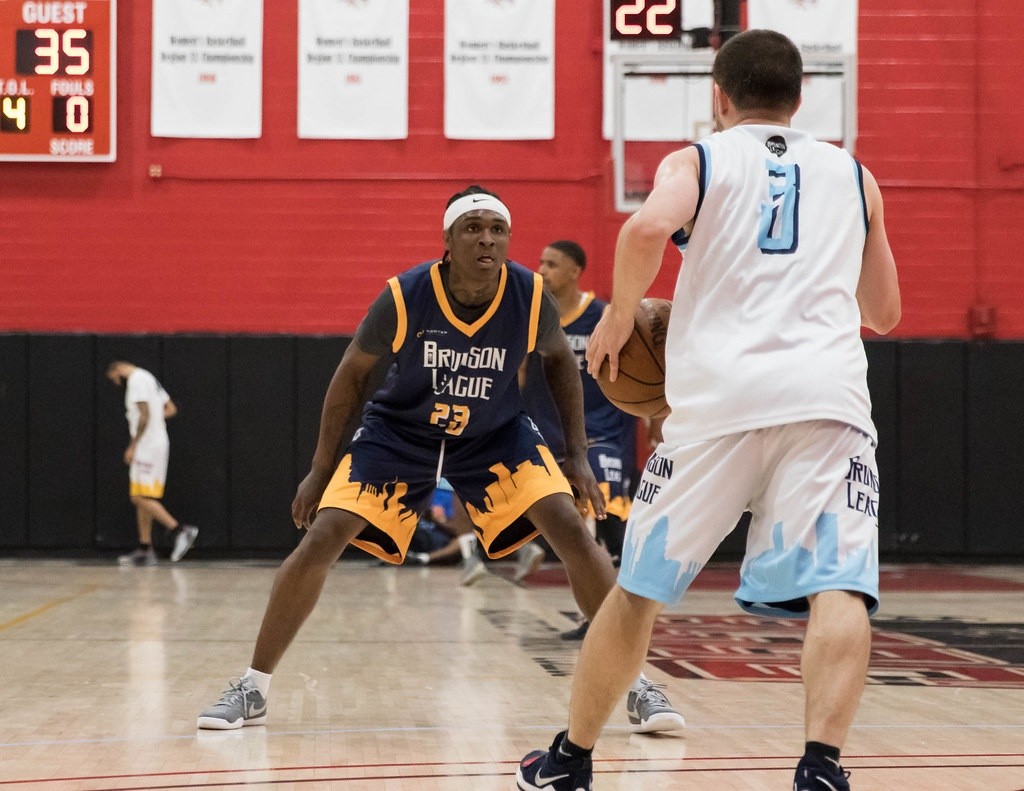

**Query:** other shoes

left=406, top=550, right=429, bottom=566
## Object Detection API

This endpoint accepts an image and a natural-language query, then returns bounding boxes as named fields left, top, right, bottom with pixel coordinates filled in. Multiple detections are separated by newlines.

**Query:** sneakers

left=627, top=680, right=685, bottom=732
left=516, top=731, right=594, bottom=791
left=197, top=675, right=267, bottom=730
left=560, top=622, right=590, bottom=641
left=118, top=544, right=158, bottom=566
left=514, top=542, right=546, bottom=581
left=459, top=556, right=487, bottom=586
left=793, top=750, right=851, bottom=791
left=170, top=523, right=198, bottom=563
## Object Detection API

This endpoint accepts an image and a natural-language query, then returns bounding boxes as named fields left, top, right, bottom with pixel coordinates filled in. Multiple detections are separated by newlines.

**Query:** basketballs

left=591, top=294, right=675, bottom=419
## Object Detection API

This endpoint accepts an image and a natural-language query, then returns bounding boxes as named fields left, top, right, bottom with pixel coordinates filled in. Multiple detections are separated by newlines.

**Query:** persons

left=516, top=29, right=902, bottom=791
left=198, top=185, right=686, bottom=733
left=105, top=360, right=197, bottom=568
